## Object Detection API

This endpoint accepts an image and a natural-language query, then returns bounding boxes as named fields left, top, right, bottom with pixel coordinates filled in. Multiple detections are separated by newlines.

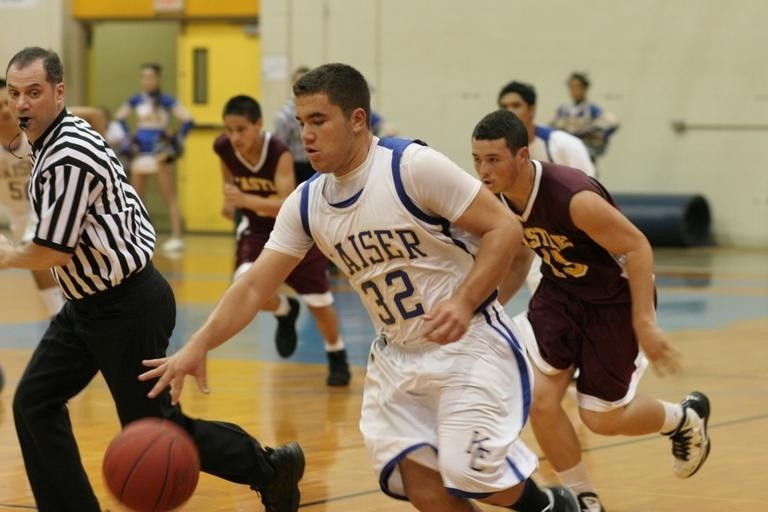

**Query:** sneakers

left=275, top=296, right=300, bottom=359
left=326, top=349, right=350, bottom=387
left=262, top=442, right=304, bottom=511
left=671, top=393, right=711, bottom=480
left=575, top=490, right=605, bottom=512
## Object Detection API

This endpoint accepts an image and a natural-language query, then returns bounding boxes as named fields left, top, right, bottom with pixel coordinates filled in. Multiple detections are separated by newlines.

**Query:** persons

left=365, top=110, right=403, bottom=142
left=1, top=79, right=108, bottom=320
left=549, top=74, right=621, bottom=166
left=210, top=91, right=351, bottom=387
left=269, top=68, right=324, bottom=188
left=136, top=61, right=581, bottom=512
left=498, top=82, right=596, bottom=179
left=469, top=108, right=711, bottom=512
left=0, top=45, right=306, bottom=512
left=104, top=59, right=194, bottom=252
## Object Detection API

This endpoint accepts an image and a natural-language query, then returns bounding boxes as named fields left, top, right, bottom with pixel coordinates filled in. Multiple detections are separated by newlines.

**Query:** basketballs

left=103, top=417, right=199, bottom=512
